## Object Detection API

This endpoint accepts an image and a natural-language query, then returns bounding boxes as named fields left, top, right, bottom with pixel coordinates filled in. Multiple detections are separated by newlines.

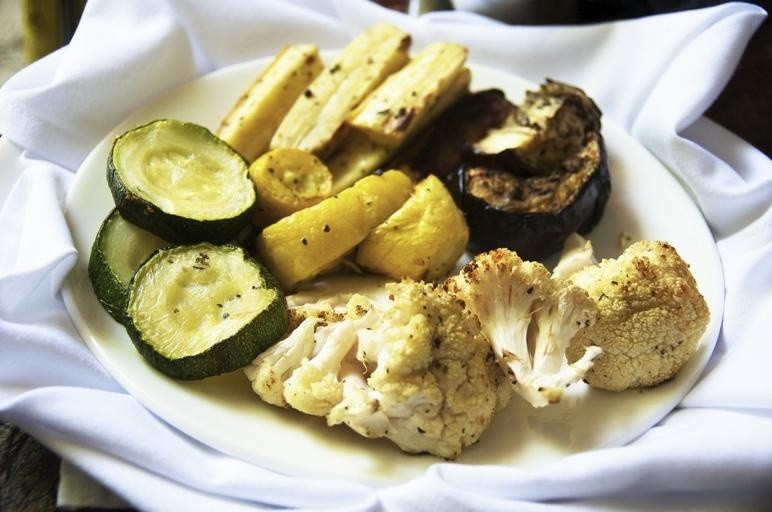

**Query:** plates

left=59, top=44, right=725, bottom=478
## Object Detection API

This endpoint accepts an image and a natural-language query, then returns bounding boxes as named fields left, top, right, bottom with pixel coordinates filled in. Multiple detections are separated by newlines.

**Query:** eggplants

left=418, top=76, right=612, bottom=262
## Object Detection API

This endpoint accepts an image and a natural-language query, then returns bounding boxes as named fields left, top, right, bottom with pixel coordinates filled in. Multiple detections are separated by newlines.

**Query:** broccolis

left=242, top=234, right=712, bottom=459
